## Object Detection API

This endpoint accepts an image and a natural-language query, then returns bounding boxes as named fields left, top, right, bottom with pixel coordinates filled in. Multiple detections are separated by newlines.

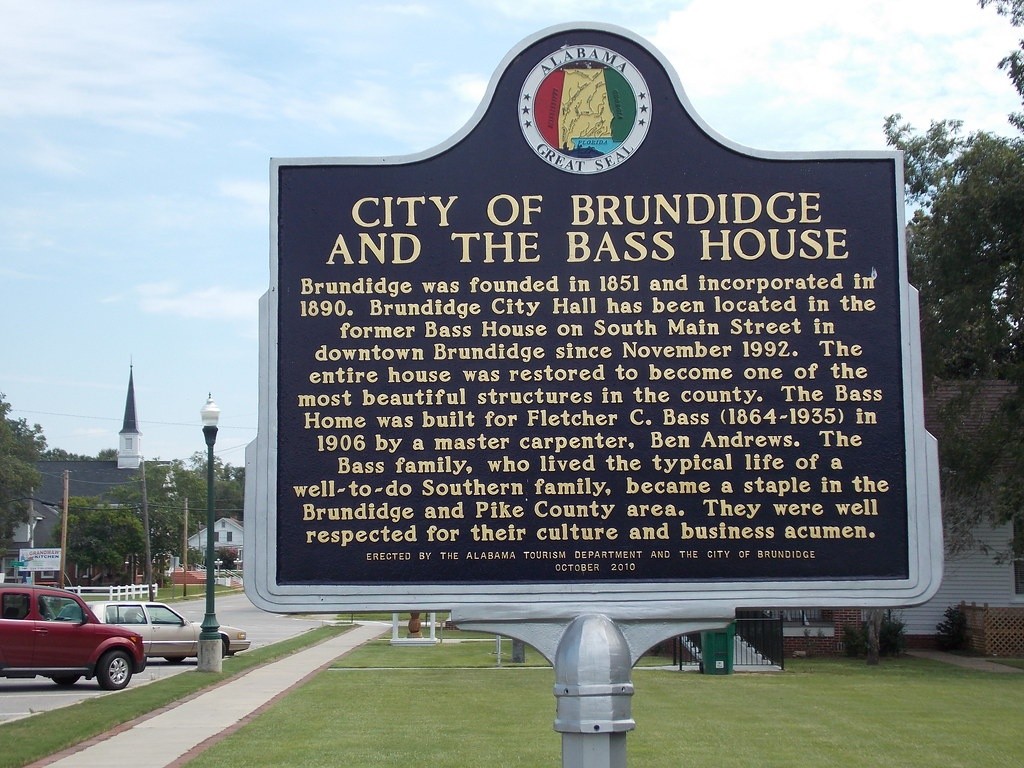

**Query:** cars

left=57, top=599, right=251, bottom=663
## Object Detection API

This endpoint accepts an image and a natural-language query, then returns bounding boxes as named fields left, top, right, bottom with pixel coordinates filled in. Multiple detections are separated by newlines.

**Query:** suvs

left=0, top=582, right=147, bottom=691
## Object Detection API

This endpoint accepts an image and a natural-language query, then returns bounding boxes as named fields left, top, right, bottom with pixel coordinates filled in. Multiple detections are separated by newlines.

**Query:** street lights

left=196, top=392, right=223, bottom=673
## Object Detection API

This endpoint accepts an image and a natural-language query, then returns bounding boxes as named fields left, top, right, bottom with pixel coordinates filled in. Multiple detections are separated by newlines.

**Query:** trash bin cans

left=698, top=620, right=738, bottom=675
